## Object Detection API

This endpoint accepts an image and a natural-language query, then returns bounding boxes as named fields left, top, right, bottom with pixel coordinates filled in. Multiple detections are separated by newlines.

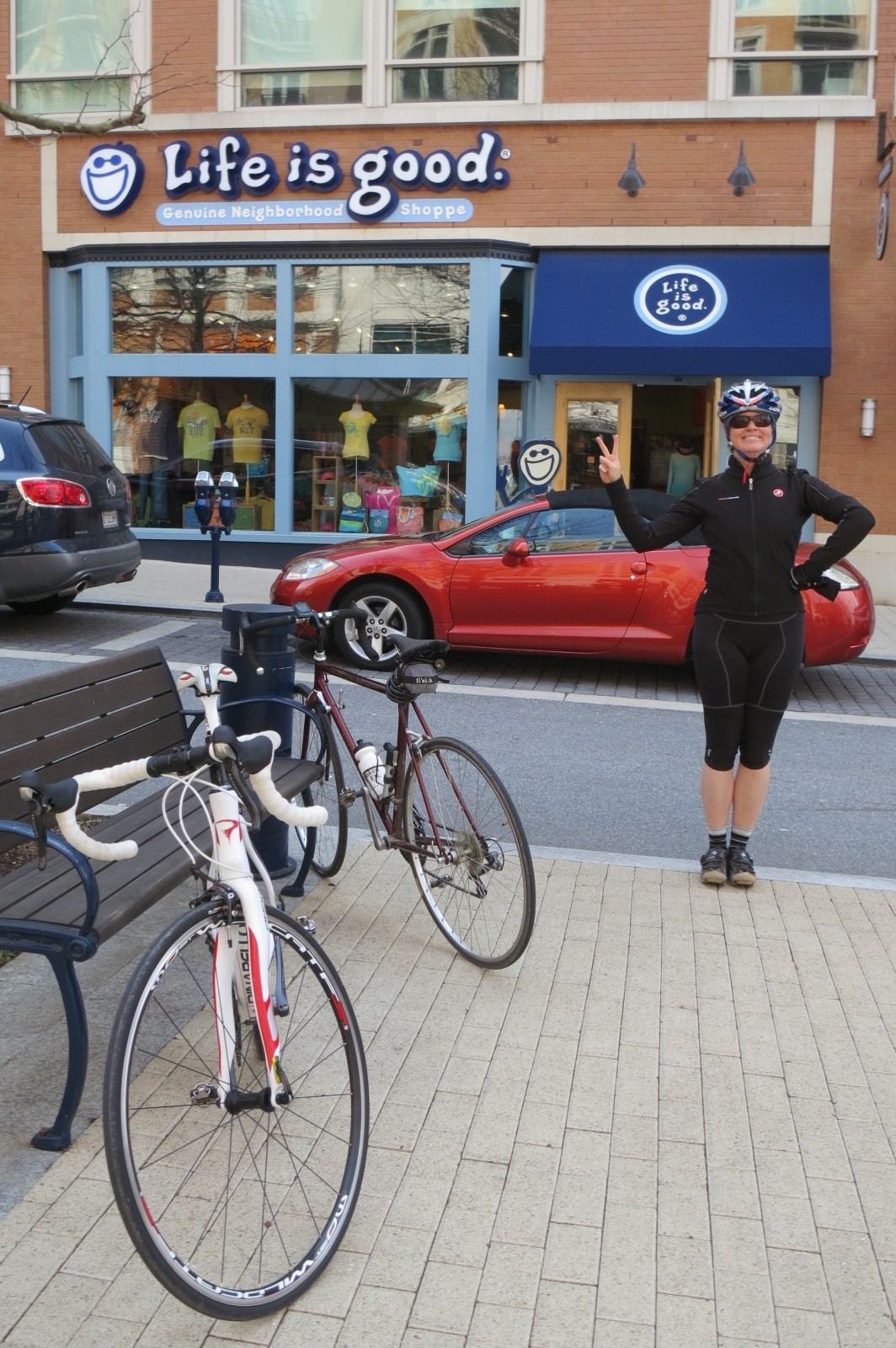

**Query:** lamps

left=860, top=398, right=877, bottom=437
left=727, top=140, right=756, bottom=196
left=0, top=366, right=12, bottom=401
left=618, top=143, right=646, bottom=197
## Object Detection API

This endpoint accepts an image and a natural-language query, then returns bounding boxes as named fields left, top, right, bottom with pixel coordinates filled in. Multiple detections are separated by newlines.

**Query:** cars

left=0, top=399, right=144, bottom=619
left=122, top=434, right=403, bottom=531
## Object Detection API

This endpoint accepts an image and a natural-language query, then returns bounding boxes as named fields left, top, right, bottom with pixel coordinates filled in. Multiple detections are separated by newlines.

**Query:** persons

left=177, top=399, right=221, bottom=460
left=132, top=385, right=175, bottom=527
left=429, top=405, right=467, bottom=462
left=339, top=403, right=377, bottom=460
left=596, top=379, right=876, bottom=885
left=224, top=402, right=269, bottom=463
left=667, top=437, right=701, bottom=497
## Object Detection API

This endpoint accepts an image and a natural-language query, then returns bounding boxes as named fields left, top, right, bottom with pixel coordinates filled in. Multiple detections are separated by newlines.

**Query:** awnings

left=529, top=249, right=832, bottom=382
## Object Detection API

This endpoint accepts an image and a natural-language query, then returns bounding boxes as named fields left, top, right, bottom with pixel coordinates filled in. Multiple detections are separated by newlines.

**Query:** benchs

left=0, top=644, right=330, bottom=1150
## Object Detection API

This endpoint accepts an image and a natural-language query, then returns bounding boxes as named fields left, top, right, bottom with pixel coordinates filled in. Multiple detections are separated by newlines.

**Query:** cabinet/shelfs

left=311, top=456, right=342, bottom=532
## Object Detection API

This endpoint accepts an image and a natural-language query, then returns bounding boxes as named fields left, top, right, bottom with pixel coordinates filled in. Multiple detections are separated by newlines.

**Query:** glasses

left=730, top=413, right=772, bottom=428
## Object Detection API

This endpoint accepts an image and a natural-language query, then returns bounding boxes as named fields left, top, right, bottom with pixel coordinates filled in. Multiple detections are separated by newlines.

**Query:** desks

left=400, top=497, right=429, bottom=533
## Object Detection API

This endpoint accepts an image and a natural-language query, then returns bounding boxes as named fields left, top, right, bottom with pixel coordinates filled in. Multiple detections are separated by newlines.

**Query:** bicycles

left=194, top=470, right=539, bottom=970
left=16, top=660, right=373, bottom=1322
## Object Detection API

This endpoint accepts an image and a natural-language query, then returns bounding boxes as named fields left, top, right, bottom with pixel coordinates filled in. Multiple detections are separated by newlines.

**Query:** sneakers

left=700, top=846, right=728, bottom=884
left=728, top=846, right=756, bottom=886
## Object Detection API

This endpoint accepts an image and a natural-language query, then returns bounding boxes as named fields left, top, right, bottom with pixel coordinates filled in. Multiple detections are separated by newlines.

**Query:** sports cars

left=266, top=483, right=876, bottom=673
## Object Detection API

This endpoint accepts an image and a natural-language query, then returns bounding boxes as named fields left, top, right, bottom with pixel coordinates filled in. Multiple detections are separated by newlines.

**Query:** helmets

left=716, top=378, right=783, bottom=422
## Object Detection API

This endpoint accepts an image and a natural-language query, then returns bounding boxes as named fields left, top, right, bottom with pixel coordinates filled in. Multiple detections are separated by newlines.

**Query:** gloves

left=812, top=575, right=841, bottom=603
left=790, top=563, right=811, bottom=593
